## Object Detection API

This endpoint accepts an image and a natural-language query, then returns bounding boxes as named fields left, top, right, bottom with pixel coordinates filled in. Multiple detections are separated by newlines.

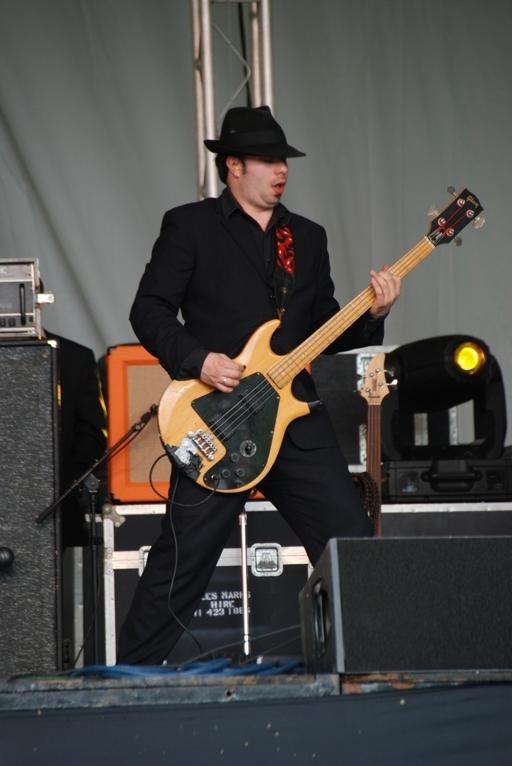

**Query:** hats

left=202, top=105, right=306, bottom=158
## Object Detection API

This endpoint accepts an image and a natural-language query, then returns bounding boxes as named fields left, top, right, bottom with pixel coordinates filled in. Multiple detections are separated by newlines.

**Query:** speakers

left=94, top=344, right=271, bottom=505
left=297, top=535, right=511, bottom=676
left=0, top=329, right=96, bottom=678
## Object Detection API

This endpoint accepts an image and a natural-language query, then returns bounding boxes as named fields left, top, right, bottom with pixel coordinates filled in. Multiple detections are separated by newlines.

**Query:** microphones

left=0, top=545, right=15, bottom=572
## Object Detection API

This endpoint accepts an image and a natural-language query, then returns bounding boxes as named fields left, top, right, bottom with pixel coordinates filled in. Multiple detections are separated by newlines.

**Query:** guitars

left=355, top=353, right=399, bottom=539
left=157, top=187, right=486, bottom=493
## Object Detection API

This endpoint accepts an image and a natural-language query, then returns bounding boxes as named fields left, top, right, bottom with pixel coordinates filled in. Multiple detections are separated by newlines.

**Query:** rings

left=222, top=376, right=227, bottom=385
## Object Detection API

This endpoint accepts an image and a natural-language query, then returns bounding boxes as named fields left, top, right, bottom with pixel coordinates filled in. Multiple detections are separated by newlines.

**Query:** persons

left=116, top=103, right=404, bottom=667
